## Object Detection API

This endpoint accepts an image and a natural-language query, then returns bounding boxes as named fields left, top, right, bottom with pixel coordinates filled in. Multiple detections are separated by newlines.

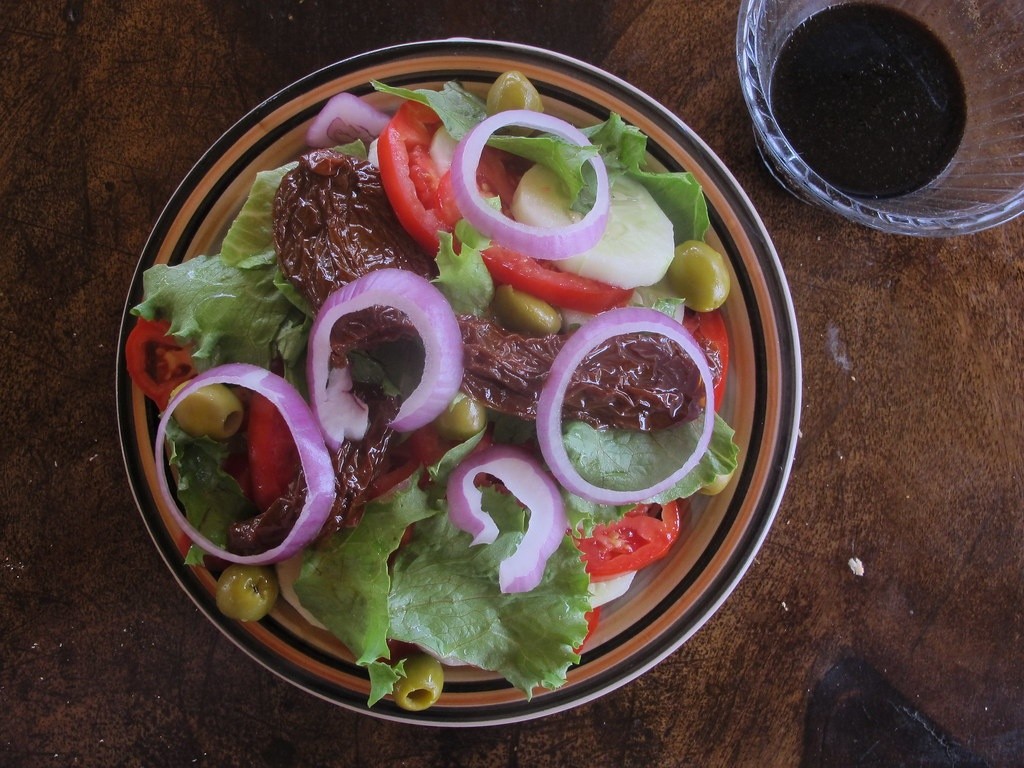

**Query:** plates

left=114, top=38, right=808, bottom=729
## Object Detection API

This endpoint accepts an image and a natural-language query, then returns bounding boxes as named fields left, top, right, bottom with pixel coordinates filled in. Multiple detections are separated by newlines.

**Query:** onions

left=447, top=108, right=611, bottom=259
left=449, top=306, right=715, bottom=594
left=155, top=269, right=464, bottom=566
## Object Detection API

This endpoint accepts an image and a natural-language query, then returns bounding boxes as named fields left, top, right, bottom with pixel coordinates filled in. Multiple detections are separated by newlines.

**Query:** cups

left=734, top=0, right=1024, bottom=237
